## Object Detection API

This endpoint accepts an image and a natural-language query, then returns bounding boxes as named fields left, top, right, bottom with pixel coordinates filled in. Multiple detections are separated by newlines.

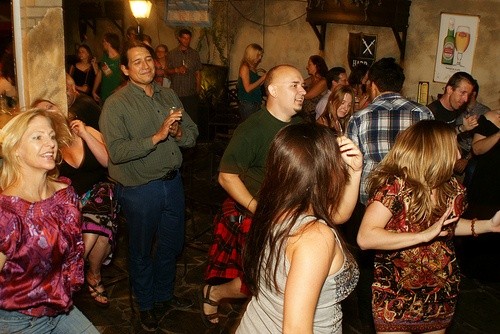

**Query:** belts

left=161, top=170, right=178, bottom=180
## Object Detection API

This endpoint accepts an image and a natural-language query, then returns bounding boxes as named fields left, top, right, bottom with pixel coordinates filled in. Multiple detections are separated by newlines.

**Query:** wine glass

left=451, top=26, right=470, bottom=67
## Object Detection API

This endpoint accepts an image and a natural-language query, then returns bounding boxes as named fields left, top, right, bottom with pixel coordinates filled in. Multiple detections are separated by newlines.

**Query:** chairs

left=184, top=164, right=222, bottom=253
left=206, top=89, right=239, bottom=140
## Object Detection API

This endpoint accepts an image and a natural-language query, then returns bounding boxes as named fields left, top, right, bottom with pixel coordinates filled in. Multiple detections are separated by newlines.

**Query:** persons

left=198, top=65, right=307, bottom=328
left=0, top=108, right=102, bottom=334
left=357, top=119, right=500, bottom=334
left=237, top=43, right=267, bottom=122
left=299, top=54, right=372, bottom=137
left=233, top=122, right=364, bottom=334
left=63, top=26, right=200, bottom=134
left=100, top=39, right=200, bottom=334
left=343, top=56, right=435, bottom=334
left=33, top=100, right=119, bottom=306
left=426, top=72, right=500, bottom=291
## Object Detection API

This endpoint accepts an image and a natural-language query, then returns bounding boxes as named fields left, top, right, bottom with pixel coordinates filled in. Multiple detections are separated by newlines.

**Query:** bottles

left=441, top=17, right=456, bottom=65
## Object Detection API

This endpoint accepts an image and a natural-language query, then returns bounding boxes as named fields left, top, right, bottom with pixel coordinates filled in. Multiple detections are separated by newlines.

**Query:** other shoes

left=135, top=298, right=159, bottom=332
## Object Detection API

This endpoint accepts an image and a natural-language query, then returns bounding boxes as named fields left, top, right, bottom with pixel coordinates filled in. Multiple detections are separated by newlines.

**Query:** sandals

left=86, top=274, right=109, bottom=307
left=200, top=284, right=220, bottom=329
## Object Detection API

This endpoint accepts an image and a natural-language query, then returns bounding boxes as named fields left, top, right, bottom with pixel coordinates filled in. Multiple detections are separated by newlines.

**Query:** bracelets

left=458, top=127, right=463, bottom=134
left=471, top=218, right=478, bottom=236
left=247, top=197, right=254, bottom=209
